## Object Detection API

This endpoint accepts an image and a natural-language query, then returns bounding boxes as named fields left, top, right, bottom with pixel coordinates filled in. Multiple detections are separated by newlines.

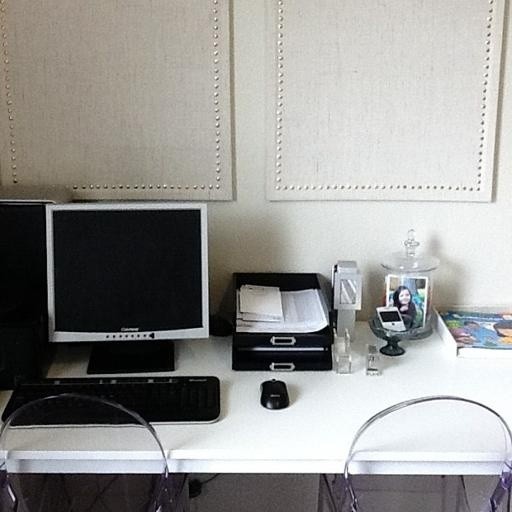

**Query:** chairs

left=0, top=393, right=190, bottom=511
left=318, top=396, right=511, bottom=512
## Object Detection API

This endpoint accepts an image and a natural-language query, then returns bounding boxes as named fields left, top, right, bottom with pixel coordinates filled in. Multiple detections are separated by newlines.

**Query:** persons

left=392, top=285, right=418, bottom=330
left=449, top=317, right=512, bottom=346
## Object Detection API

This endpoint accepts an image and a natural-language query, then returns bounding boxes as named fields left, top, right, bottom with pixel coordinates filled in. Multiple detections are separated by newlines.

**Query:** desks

left=0, top=317, right=512, bottom=512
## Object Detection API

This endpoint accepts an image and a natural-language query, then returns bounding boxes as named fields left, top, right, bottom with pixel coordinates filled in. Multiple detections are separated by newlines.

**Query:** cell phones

left=376, top=306, right=407, bottom=332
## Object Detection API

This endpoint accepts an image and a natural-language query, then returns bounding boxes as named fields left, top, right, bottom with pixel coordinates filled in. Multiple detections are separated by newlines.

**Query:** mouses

left=259, top=379, right=289, bottom=410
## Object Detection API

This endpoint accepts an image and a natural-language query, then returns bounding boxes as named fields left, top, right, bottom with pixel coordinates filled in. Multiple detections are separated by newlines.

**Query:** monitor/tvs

left=45, top=203, right=209, bottom=374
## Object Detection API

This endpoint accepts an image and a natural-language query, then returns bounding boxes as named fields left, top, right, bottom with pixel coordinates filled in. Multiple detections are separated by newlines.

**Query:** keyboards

left=3, top=376, right=221, bottom=429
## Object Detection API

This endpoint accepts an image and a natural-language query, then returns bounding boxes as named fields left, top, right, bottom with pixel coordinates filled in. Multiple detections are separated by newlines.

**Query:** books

left=435, top=309, right=512, bottom=360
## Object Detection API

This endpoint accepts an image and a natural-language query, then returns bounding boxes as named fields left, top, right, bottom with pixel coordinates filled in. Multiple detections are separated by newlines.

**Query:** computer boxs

left=0, top=199, right=54, bottom=390
